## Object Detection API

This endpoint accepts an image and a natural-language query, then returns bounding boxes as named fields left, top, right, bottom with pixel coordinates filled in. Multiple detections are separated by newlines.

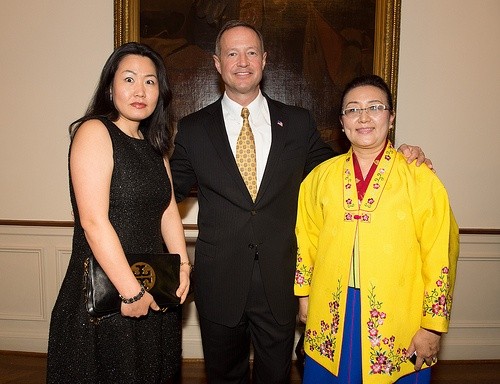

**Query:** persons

left=46, top=43, right=191, bottom=384
left=294, top=74, right=460, bottom=384
left=169, top=21, right=433, bottom=384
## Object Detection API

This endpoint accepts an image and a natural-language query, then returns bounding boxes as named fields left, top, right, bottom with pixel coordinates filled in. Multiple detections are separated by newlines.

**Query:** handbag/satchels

left=83, top=252, right=181, bottom=325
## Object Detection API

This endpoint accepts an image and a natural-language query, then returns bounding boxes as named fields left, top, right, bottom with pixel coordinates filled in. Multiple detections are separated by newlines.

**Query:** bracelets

left=180, top=262, right=192, bottom=271
left=119, top=285, right=145, bottom=304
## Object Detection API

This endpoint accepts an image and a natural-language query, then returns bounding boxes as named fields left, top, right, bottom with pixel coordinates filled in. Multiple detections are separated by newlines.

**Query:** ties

left=236, top=108, right=257, bottom=204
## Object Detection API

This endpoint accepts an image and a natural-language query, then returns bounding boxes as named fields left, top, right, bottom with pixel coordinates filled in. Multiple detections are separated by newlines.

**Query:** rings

left=433, top=356, right=435, bottom=362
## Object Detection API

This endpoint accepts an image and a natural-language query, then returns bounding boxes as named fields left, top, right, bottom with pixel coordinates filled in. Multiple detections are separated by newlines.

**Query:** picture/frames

left=113, top=0, right=401, bottom=197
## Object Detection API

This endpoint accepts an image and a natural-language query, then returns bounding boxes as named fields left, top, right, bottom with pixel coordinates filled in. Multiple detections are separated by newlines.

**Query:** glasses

left=341, top=104, right=389, bottom=118
